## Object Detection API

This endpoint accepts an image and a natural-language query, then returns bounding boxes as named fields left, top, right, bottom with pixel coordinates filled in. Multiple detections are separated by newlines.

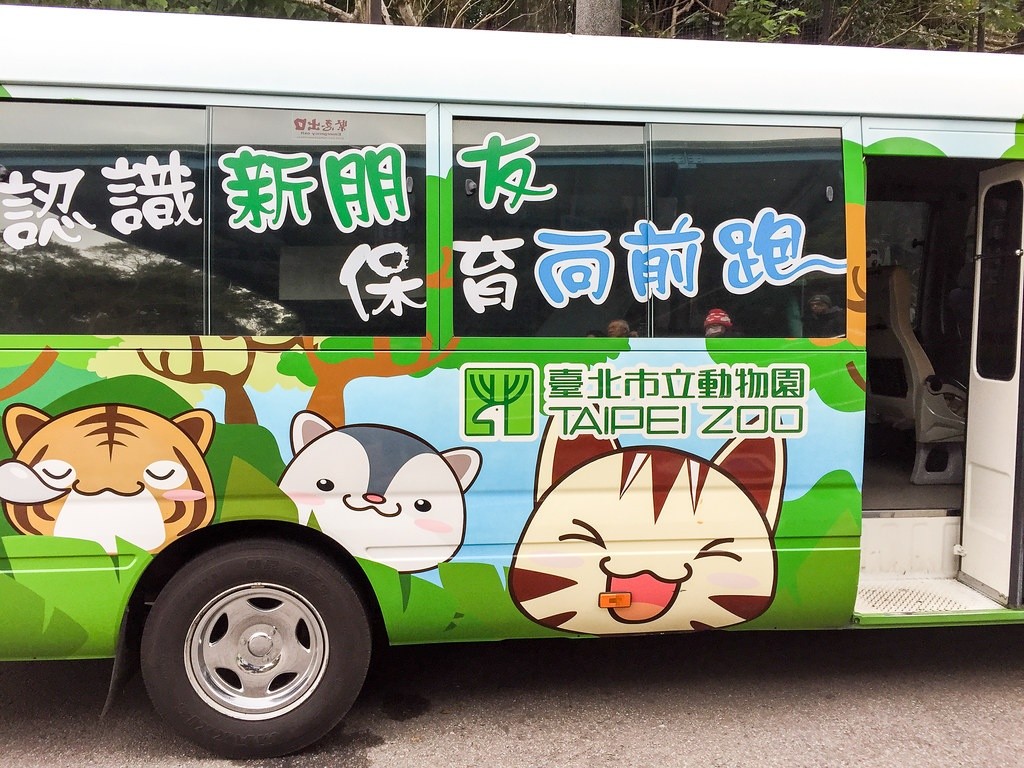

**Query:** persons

left=587, top=319, right=638, bottom=337
left=801, top=294, right=847, bottom=338
left=704, top=308, right=745, bottom=338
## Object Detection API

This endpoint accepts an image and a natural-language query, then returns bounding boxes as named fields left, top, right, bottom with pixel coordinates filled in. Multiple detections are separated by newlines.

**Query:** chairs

left=950, top=263, right=974, bottom=344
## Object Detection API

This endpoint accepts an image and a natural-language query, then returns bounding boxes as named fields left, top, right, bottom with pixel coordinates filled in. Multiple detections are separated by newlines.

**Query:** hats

left=704, top=309, right=732, bottom=328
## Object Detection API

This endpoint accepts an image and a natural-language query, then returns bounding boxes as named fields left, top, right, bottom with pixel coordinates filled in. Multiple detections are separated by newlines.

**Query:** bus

left=1, top=6, right=1024, bottom=761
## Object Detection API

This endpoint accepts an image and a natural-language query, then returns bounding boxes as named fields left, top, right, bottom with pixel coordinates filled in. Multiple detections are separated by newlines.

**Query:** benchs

left=867, top=265, right=968, bottom=485
